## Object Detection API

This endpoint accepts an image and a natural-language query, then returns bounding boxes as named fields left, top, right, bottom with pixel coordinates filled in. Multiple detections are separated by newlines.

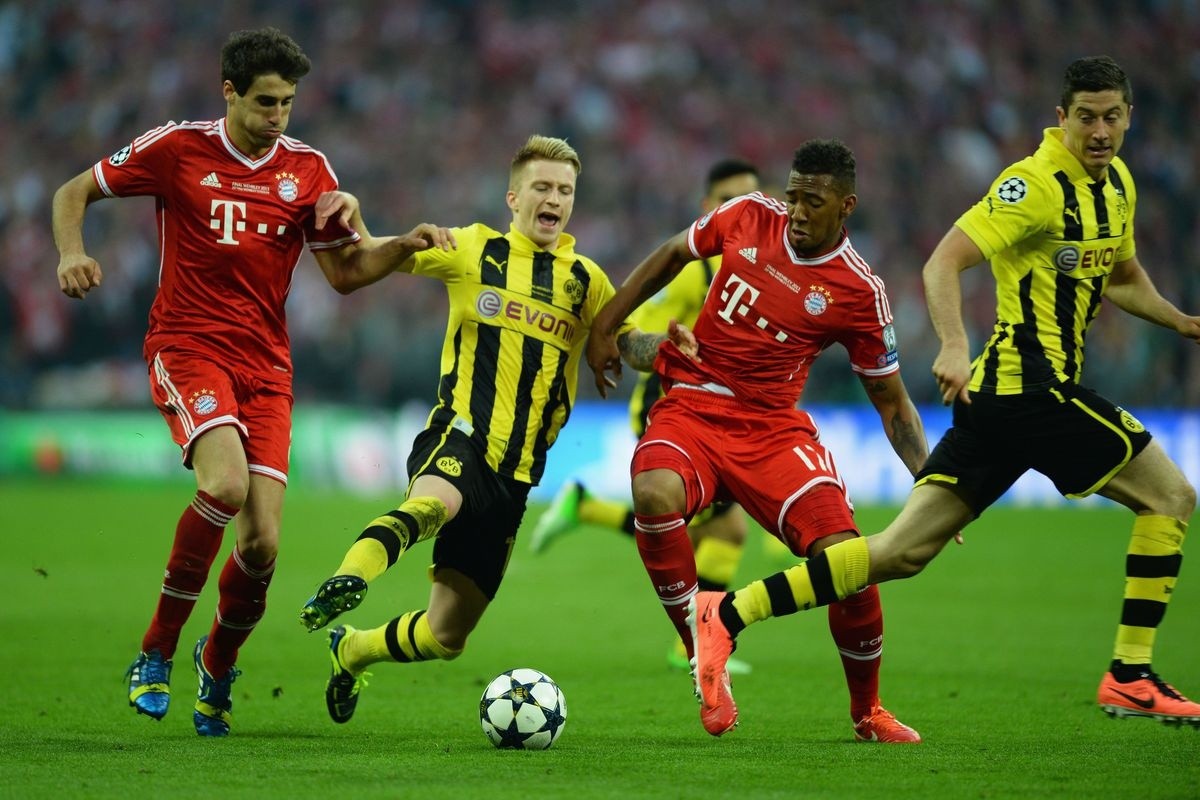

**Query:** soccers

left=479, top=667, right=569, bottom=753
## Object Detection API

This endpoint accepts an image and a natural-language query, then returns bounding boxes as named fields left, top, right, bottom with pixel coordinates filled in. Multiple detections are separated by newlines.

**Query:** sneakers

left=298, top=575, right=369, bottom=634
left=191, top=635, right=242, bottom=737
left=851, top=704, right=922, bottom=745
left=694, top=667, right=741, bottom=738
left=683, top=589, right=736, bottom=710
left=121, top=646, right=174, bottom=722
left=1096, top=658, right=1200, bottom=726
left=326, top=623, right=374, bottom=723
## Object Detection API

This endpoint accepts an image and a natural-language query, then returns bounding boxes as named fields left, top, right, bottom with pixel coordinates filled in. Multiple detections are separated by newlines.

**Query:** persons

left=586, top=138, right=963, bottom=745
left=684, top=55, right=1200, bottom=730
left=51, top=23, right=458, bottom=736
left=527, top=157, right=759, bottom=676
left=299, top=134, right=698, bottom=723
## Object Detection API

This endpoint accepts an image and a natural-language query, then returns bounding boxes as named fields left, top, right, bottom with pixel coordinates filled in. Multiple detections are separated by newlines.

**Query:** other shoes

left=531, top=475, right=589, bottom=554
left=667, top=644, right=753, bottom=676
